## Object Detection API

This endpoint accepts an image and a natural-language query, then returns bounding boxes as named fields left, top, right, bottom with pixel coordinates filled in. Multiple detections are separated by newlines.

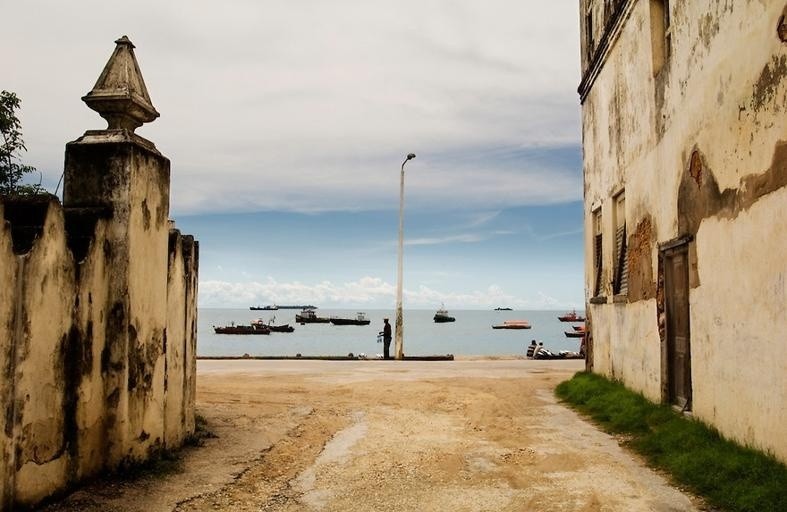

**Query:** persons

left=532, top=341, right=545, bottom=360
left=526, top=339, right=537, bottom=357
left=376, top=317, right=392, bottom=358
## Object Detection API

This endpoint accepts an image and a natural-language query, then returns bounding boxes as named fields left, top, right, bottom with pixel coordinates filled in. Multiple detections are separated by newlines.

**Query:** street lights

left=393, top=153, right=419, bottom=361
